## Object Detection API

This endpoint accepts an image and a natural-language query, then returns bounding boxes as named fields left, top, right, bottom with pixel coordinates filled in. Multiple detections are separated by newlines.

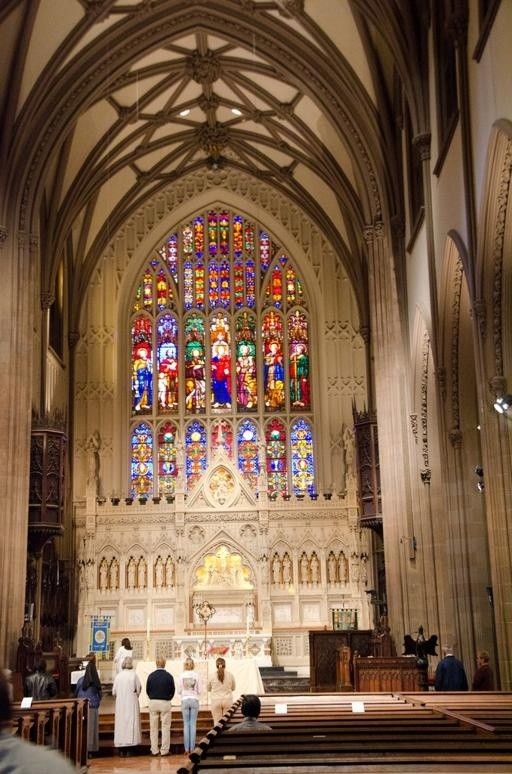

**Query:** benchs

left=0, top=698, right=89, bottom=774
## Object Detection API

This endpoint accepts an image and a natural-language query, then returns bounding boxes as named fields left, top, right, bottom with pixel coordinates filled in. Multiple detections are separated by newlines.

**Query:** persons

left=435, top=644, right=468, bottom=691
left=207, top=658, right=235, bottom=726
left=23, top=659, right=57, bottom=699
left=131, top=342, right=308, bottom=412
left=0, top=669, right=74, bottom=773
left=146, top=655, right=176, bottom=756
left=74, top=662, right=102, bottom=759
left=113, top=638, right=133, bottom=673
left=473, top=651, right=494, bottom=690
left=228, top=695, right=273, bottom=731
left=112, top=656, right=142, bottom=757
left=177, top=658, right=202, bottom=755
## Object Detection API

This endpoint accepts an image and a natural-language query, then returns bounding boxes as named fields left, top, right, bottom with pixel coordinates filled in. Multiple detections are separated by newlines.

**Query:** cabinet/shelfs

left=353, top=656, right=421, bottom=692
left=308, top=630, right=375, bottom=692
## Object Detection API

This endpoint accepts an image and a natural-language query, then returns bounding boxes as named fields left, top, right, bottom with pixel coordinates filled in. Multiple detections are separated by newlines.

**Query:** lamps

left=493, top=394, right=512, bottom=414
left=474, top=465, right=484, bottom=493
